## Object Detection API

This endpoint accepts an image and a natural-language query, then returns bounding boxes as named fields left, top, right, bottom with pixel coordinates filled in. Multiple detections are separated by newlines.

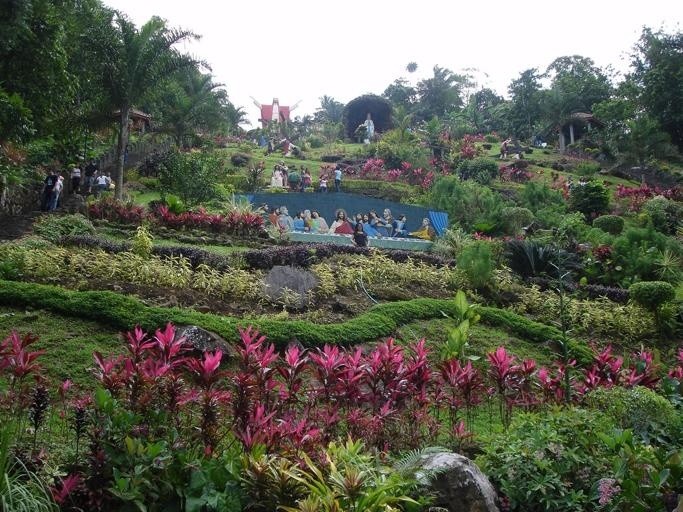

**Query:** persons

left=247, top=159, right=346, bottom=194
left=36, top=153, right=115, bottom=214
left=498, top=137, right=512, bottom=160
left=350, top=222, right=369, bottom=249
left=358, top=111, right=374, bottom=145
left=250, top=133, right=297, bottom=159
left=250, top=201, right=438, bottom=241
